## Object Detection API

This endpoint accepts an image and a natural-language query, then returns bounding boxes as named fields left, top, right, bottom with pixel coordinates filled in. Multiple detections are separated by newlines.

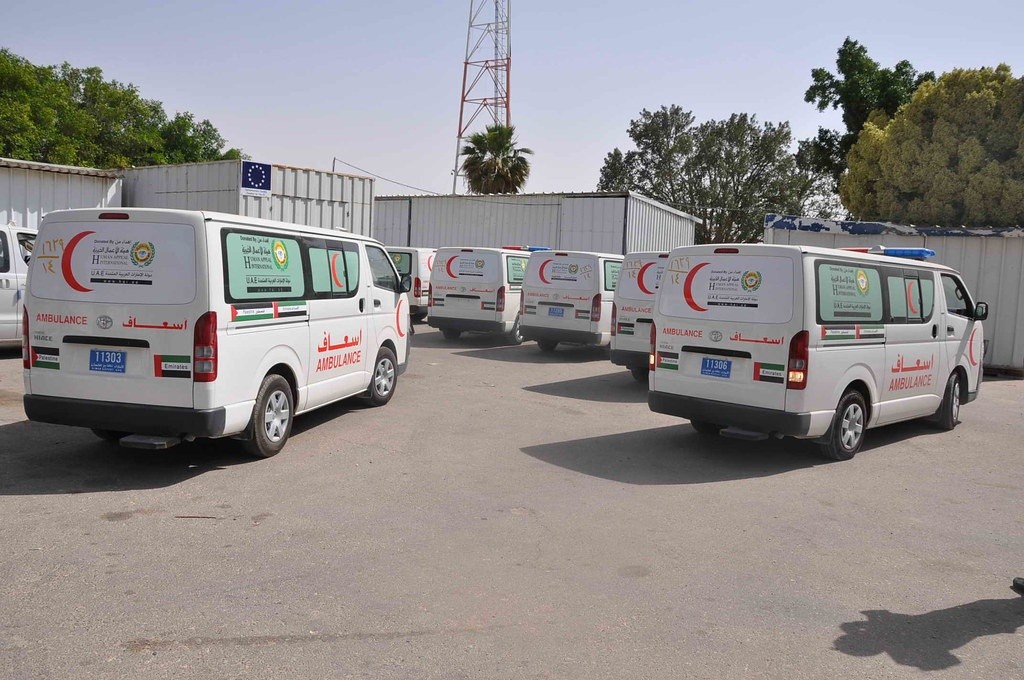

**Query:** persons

left=1012, top=577, right=1024, bottom=591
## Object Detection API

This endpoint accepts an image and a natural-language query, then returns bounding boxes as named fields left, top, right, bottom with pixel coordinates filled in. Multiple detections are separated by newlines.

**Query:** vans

left=519, top=249, right=627, bottom=353
left=0, top=225, right=41, bottom=351
left=20, top=205, right=411, bottom=460
left=425, top=244, right=555, bottom=346
left=375, top=245, right=444, bottom=321
left=646, top=239, right=989, bottom=462
left=609, top=252, right=670, bottom=382
